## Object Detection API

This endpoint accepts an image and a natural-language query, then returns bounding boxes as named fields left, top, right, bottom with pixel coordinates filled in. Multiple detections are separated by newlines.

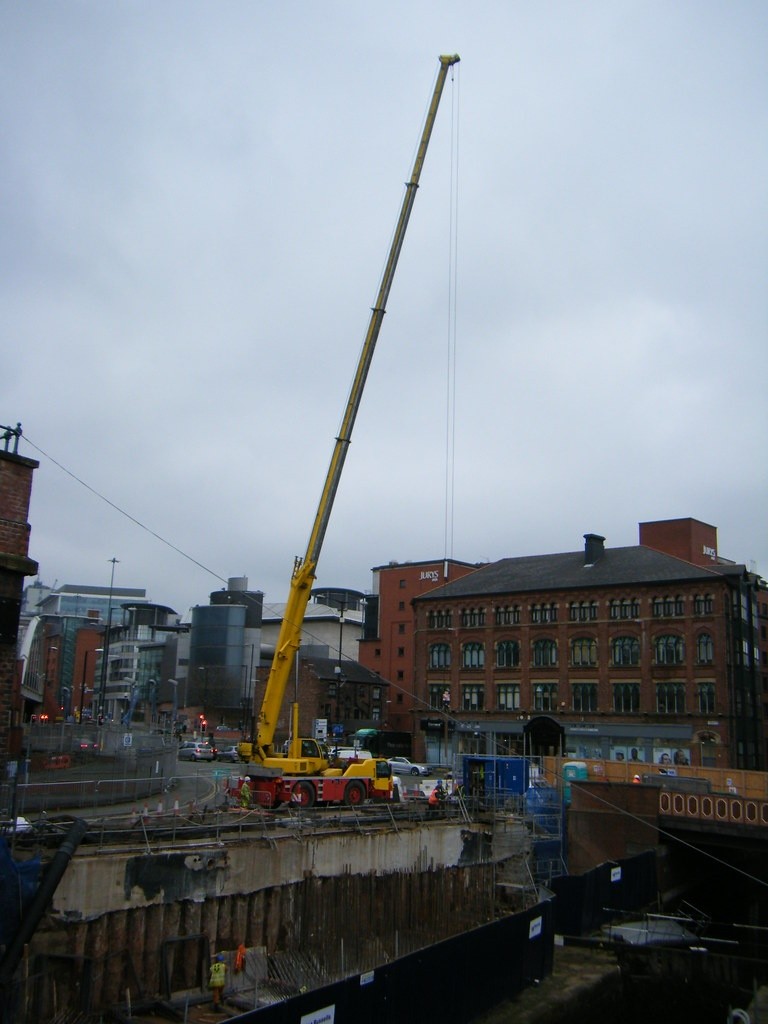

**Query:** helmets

left=217, top=955, right=224, bottom=961
left=244, top=776, right=250, bottom=781
left=437, top=780, right=442, bottom=783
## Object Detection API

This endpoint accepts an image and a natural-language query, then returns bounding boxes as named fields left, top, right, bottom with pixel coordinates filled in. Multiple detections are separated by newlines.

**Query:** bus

left=348, top=728, right=413, bottom=761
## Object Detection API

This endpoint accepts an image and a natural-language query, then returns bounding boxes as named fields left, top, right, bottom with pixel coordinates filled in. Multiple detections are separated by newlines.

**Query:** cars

left=282, top=739, right=292, bottom=752
left=5, top=817, right=35, bottom=837
left=71, top=737, right=99, bottom=754
left=387, top=756, right=434, bottom=776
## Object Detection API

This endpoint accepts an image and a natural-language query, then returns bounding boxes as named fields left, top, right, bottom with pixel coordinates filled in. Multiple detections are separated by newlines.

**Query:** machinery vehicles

left=222, top=54, right=461, bottom=812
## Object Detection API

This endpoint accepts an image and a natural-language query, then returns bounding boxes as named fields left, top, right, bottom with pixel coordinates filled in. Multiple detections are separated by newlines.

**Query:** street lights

left=79, top=649, right=104, bottom=724
left=102, top=606, right=138, bottom=726
left=40, top=646, right=59, bottom=724
left=99, top=558, right=120, bottom=725
left=184, top=666, right=205, bottom=737
left=316, top=594, right=370, bottom=756
left=169, top=679, right=178, bottom=730
left=150, top=679, right=157, bottom=733
left=242, top=642, right=254, bottom=745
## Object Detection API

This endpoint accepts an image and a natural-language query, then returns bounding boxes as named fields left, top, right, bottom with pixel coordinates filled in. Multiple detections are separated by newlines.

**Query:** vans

left=330, top=750, right=372, bottom=759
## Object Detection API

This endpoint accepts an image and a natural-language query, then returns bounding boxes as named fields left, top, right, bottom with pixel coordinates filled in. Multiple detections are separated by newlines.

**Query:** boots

left=214, top=1004, right=220, bottom=1012
left=220, top=999, right=224, bottom=1005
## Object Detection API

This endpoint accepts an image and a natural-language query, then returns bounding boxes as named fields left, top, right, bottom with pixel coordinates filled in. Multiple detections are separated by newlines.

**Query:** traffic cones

left=131, top=807, right=137, bottom=827
left=401, top=781, right=408, bottom=799
left=170, top=796, right=181, bottom=817
left=413, top=781, right=419, bottom=797
left=141, top=803, right=149, bottom=824
left=156, top=800, right=166, bottom=823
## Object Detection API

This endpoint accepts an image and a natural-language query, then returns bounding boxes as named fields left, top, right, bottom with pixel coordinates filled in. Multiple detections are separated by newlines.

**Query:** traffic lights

left=31, top=714, right=36, bottom=727
left=60, top=687, right=70, bottom=712
left=39, top=714, right=44, bottom=727
left=44, top=714, right=48, bottom=727
left=199, top=714, right=204, bottom=720
left=200, top=720, right=206, bottom=733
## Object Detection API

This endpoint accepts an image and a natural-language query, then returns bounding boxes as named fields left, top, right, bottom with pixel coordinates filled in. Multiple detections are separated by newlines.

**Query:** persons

left=659, top=753, right=671, bottom=765
left=615, top=749, right=625, bottom=761
left=633, top=774, right=640, bottom=783
left=629, top=748, right=643, bottom=762
left=677, top=750, right=689, bottom=765
left=208, top=954, right=229, bottom=1011
left=240, top=776, right=253, bottom=810
left=428, top=780, right=447, bottom=815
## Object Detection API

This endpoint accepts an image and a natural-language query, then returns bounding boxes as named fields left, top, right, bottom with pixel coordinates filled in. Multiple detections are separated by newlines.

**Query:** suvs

left=216, top=747, right=239, bottom=762
left=178, top=741, right=214, bottom=761
